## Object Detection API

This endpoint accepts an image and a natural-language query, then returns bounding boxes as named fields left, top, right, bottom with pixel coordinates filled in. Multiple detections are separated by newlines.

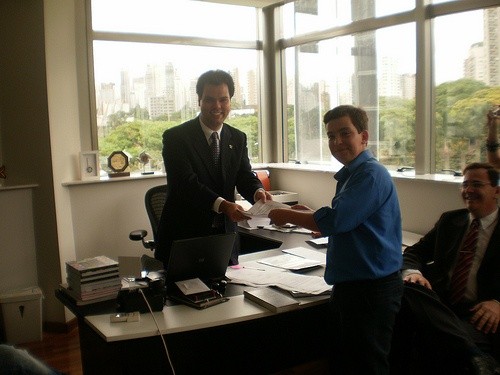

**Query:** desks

left=54, top=215, right=426, bottom=375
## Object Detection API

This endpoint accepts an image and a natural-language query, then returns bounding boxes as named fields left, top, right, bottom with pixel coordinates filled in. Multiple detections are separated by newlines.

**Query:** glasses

left=460, top=181, right=491, bottom=189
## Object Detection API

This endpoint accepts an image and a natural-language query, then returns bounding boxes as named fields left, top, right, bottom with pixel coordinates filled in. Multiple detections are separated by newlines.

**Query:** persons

left=154, top=71, right=272, bottom=265
left=268, top=105, right=404, bottom=375
left=400, top=163, right=500, bottom=375
left=487, top=109, right=500, bottom=169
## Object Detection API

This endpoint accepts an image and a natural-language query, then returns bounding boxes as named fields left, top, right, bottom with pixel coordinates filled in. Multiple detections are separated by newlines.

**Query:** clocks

left=107, top=151, right=129, bottom=172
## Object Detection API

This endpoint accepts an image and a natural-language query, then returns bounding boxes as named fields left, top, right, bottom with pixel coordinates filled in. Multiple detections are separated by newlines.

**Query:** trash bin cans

left=0, top=284, right=42, bottom=346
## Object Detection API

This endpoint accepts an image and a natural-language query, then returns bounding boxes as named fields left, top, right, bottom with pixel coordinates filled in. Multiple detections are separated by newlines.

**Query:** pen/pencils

left=196, top=298, right=230, bottom=311
left=242, top=267, right=267, bottom=271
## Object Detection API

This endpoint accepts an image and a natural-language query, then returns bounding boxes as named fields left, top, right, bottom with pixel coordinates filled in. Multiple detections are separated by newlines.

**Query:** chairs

left=128, top=185, right=240, bottom=272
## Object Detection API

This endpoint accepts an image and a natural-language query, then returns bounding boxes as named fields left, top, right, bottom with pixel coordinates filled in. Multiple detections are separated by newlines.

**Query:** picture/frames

left=79, top=150, right=100, bottom=181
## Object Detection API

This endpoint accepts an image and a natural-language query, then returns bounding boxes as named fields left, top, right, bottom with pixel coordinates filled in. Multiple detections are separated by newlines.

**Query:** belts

left=381, top=269, right=401, bottom=280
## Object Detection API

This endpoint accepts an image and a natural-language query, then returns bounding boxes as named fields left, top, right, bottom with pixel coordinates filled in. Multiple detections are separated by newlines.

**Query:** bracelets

left=486, top=141, right=500, bottom=152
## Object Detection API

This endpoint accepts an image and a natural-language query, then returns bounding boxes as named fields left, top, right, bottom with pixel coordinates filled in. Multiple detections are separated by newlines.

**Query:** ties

left=445, top=218, right=481, bottom=312
left=208, top=131, right=222, bottom=177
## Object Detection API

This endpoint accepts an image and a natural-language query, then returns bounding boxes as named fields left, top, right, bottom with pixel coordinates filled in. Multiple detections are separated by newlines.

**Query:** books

left=243, top=287, right=299, bottom=314
left=59, top=255, right=123, bottom=306
left=290, top=276, right=333, bottom=298
